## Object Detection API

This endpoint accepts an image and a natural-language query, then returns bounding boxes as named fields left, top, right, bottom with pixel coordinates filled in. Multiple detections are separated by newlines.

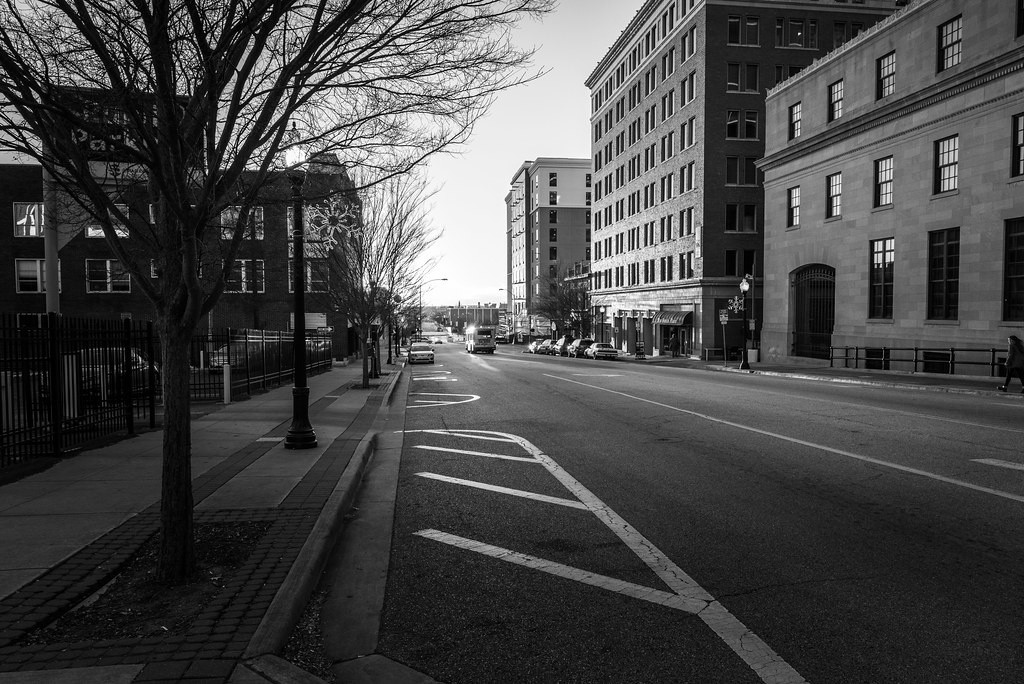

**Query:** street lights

left=498, top=287, right=518, bottom=344
left=392, top=277, right=449, bottom=357
left=281, top=123, right=321, bottom=451
left=598, top=306, right=605, bottom=344
left=739, top=279, right=752, bottom=369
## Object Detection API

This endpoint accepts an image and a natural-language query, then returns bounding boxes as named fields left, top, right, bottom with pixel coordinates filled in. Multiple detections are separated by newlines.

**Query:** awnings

left=651, top=311, right=693, bottom=326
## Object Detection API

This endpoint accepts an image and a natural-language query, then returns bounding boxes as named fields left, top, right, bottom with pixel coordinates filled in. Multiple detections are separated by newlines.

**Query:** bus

left=465, top=327, right=495, bottom=353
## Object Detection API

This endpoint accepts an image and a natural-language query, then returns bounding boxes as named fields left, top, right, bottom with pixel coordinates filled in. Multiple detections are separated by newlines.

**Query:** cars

left=552, top=335, right=575, bottom=356
left=526, top=339, right=554, bottom=354
left=408, top=342, right=435, bottom=364
left=569, top=339, right=593, bottom=356
left=584, top=341, right=618, bottom=360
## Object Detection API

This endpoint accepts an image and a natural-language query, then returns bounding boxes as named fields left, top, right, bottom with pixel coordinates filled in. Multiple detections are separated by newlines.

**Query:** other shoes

left=997, top=386, right=1007, bottom=392
left=1021, top=388, right=1024, bottom=393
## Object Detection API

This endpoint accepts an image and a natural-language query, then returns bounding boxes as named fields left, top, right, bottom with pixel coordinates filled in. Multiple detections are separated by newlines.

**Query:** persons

left=669, top=334, right=680, bottom=359
left=996, top=335, right=1024, bottom=393
